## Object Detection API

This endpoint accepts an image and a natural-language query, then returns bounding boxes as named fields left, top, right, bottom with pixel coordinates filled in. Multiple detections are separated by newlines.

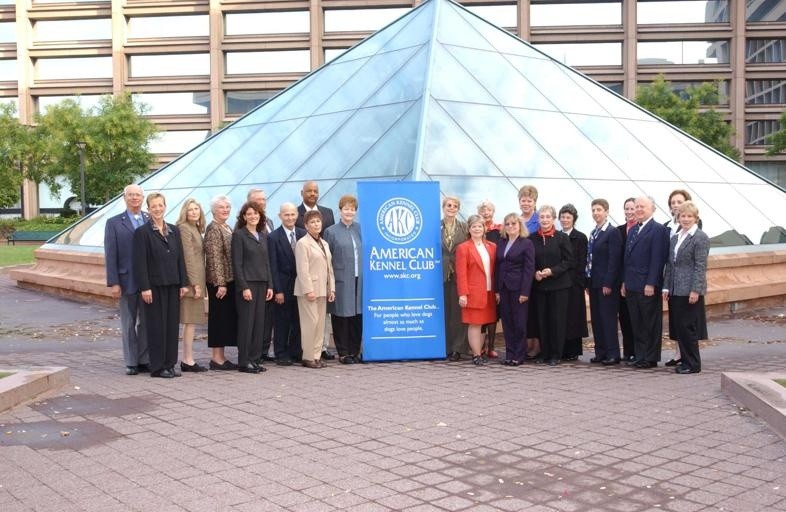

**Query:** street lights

left=74, top=138, right=87, bottom=219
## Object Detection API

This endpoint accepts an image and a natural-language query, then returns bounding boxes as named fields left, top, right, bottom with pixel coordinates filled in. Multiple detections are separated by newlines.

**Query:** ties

left=289, top=232, right=296, bottom=255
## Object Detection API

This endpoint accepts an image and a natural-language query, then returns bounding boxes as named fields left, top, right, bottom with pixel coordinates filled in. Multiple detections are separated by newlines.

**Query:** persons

left=174, top=198, right=210, bottom=373
left=586, top=199, right=626, bottom=365
left=230, top=181, right=363, bottom=374
left=620, top=194, right=669, bottom=369
left=441, top=185, right=590, bottom=367
left=661, top=201, right=711, bottom=374
left=202, top=194, right=239, bottom=371
left=614, top=197, right=649, bottom=362
left=102, top=183, right=157, bottom=375
left=132, top=193, right=190, bottom=377
left=659, top=190, right=705, bottom=366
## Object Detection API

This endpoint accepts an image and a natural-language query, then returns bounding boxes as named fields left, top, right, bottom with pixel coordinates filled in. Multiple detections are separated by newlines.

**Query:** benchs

left=6, top=231, right=62, bottom=246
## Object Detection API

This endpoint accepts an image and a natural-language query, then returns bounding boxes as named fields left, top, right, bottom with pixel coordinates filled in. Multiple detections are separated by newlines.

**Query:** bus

left=0, top=160, right=138, bottom=218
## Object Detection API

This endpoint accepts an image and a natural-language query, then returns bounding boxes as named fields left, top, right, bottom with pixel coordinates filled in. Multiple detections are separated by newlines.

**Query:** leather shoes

left=125, top=350, right=359, bottom=378
left=449, top=349, right=701, bottom=373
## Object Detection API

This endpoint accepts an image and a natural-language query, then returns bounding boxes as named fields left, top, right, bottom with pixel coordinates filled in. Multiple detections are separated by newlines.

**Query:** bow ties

left=637, top=222, right=643, bottom=226
